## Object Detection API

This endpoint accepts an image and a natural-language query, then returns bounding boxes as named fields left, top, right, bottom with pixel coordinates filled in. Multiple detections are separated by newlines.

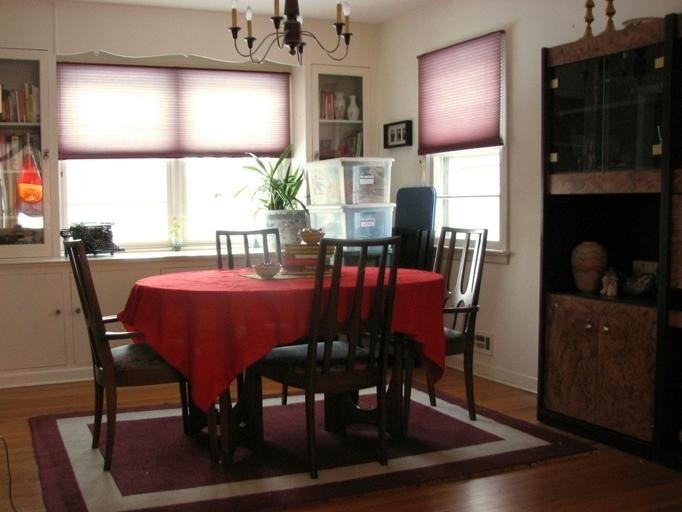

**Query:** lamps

left=225, top=0, right=354, bottom=68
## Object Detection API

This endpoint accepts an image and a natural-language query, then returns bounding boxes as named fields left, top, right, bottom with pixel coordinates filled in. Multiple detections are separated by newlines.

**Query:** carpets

left=27, top=380, right=600, bottom=512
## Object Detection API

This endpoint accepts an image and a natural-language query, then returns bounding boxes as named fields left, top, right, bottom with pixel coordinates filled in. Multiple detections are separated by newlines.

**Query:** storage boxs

left=307, top=203, right=396, bottom=251
left=301, top=156, right=396, bottom=205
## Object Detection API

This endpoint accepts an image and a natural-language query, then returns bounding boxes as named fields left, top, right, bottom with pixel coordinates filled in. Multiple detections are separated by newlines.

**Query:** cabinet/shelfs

left=290, top=65, right=368, bottom=206
left=533, top=13, right=682, bottom=303
left=537, top=287, right=682, bottom=462
left=1, top=48, right=62, bottom=258
left=0, top=261, right=160, bottom=375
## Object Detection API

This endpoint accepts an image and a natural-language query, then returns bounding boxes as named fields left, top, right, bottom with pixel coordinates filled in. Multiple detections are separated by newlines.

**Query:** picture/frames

left=383, top=120, right=412, bottom=149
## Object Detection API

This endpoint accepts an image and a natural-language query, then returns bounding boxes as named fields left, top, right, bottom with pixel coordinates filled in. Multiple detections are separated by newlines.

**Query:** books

left=0, top=82, right=40, bottom=172
left=319, top=89, right=335, bottom=120
left=281, top=244, right=335, bottom=275
left=346, top=130, right=362, bottom=158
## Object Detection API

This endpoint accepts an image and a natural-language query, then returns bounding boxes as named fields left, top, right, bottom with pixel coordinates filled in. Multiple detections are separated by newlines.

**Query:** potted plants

left=230, top=144, right=314, bottom=246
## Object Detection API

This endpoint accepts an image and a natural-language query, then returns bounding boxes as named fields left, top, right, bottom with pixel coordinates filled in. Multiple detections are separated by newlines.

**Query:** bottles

left=333, top=91, right=347, bottom=120
left=570, top=240, right=608, bottom=292
left=346, top=94, right=360, bottom=121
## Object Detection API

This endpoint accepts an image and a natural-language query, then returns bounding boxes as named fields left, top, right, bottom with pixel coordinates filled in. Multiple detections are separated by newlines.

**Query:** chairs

left=244, top=233, right=407, bottom=481
left=380, top=225, right=490, bottom=420
left=216, top=228, right=292, bottom=409
left=392, top=186, right=439, bottom=272
left=62, top=234, right=222, bottom=471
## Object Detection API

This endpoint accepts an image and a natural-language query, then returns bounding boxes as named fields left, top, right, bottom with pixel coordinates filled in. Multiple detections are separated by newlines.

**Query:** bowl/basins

left=300, top=230, right=324, bottom=245
left=254, top=265, right=280, bottom=279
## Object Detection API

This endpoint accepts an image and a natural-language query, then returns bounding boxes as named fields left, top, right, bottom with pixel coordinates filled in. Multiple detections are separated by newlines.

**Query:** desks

left=116, top=264, right=447, bottom=453
